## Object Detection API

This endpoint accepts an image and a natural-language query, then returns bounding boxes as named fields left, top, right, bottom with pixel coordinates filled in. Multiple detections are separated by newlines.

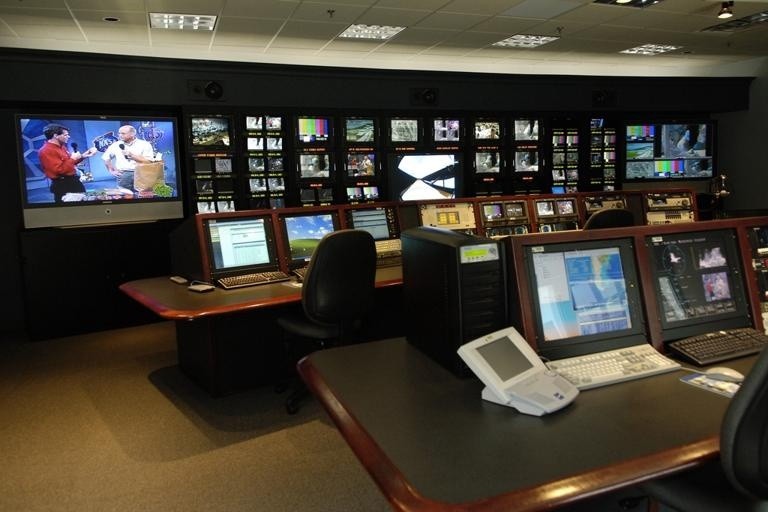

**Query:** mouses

left=705, top=364, right=745, bottom=383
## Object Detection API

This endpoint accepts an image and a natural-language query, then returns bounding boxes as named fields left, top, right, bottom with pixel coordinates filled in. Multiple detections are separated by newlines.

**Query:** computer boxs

left=401, top=226, right=506, bottom=381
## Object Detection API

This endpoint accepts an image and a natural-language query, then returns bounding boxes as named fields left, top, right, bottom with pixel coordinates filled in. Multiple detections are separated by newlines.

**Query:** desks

left=118, top=268, right=415, bottom=397
left=284, top=322, right=768, bottom=510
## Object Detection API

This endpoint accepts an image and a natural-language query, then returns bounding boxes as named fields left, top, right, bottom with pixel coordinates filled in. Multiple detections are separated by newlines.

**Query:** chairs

left=272, top=225, right=384, bottom=423
left=582, top=206, right=643, bottom=232
left=628, top=311, right=767, bottom=511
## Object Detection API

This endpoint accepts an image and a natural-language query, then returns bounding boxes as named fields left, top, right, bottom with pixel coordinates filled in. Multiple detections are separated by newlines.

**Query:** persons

left=38, top=124, right=98, bottom=202
left=101, top=124, right=154, bottom=190
left=706, top=274, right=725, bottom=300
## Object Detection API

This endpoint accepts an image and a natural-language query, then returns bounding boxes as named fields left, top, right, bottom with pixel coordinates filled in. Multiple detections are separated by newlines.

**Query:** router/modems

left=187, top=284, right=215, bottom=293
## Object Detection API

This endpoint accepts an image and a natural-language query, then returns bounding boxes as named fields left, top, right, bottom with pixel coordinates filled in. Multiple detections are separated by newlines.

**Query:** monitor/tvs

left=276, top=205, right=344, bottom=275
left=188, top=113, right=713, bottom=238
left=745, top=217, right=768, bottom=328
left=195, top=210, right=290, bottom=283
left=512, top=228, right=650, bottom=359
left=15, top=110, right=184, bottom=230
left=639, top=219, right=763, bottom=351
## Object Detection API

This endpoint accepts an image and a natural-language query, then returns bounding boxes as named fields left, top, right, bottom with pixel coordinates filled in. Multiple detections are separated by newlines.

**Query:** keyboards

left=547, top=343, right=683, bottom=392
left=218, top=272, right=290, bottom=289
left=671, top=328, right=768, bottom=365
left=376, top=256, right=401, bottom=269
left=293, top=268, right=307, bottom=282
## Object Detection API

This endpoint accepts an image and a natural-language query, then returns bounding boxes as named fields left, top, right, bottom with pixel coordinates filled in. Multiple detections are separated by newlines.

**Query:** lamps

left=714, top=0, right=743, bottom=23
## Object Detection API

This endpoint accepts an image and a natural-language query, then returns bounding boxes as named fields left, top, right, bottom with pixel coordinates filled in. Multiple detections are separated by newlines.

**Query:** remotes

left=170, top=276, right=187, bottom=284
